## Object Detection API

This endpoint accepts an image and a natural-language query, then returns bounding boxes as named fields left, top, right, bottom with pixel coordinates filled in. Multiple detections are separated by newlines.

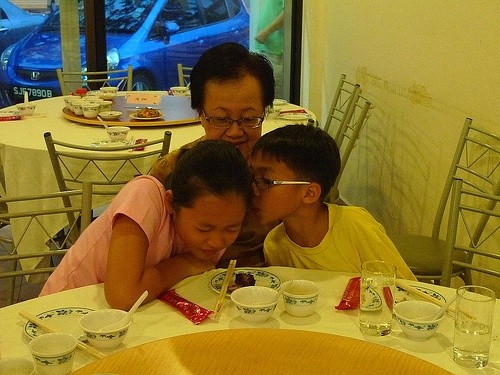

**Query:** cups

left=269, top=108, right=281, bottom=120
left=28, top=332, right=77, bottom=375
left=359, top=260, right=397, bottom=336
left=0, top=356, right=36, bottom=375
left=453, top=285, right=496, bottom=368
left=281, top=280, right=320, bottom=316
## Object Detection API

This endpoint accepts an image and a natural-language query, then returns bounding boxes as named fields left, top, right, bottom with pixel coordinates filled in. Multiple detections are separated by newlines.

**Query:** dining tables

left=0, top=91, right=318, bottom=282
left=0, top=267, right=500, bottom=375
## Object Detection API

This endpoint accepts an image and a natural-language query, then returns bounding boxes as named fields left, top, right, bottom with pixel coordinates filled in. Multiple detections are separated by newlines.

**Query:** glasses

left=201, top=106, right=266, bottom=130
left=251, top=173, right=311, bottom=190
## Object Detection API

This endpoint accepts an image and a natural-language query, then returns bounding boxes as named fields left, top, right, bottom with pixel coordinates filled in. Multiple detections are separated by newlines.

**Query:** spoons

left=100, top=291, right=148, bottom=331
left=410, top=287, right=466, bottom=321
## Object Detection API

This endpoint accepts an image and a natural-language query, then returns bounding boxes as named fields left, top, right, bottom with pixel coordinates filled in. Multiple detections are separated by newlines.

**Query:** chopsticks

left=397, top=281, right=476, bottom=324
left=208, top=260, right=236, bottom=320
left=19, top=310, right=106, bottom=360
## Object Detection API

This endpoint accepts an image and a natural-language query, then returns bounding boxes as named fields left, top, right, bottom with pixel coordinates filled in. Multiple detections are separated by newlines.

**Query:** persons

left=250, top=0, right=284, bottom=99
left=39, top=139, right=251, bottom=312
left=251, top=124, right=417, bottom=281
left=150, top=42, right=282, bottom=269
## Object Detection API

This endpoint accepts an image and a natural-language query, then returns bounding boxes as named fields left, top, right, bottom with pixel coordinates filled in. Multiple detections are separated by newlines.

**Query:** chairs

left=0, top=63, right=500, bottom=306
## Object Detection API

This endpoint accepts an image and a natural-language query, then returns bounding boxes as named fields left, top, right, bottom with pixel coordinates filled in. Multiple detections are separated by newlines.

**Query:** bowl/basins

left=171, top=87, right=189, bottom=95
left=15, top=87, right=120, bottom=118
left=230, top=286, right=278, bottom=323
left=104, top=126, right=130, bottom=141
left=392, top=301, right=446, bottom=337
left=79, top=309, right=133, bottom=348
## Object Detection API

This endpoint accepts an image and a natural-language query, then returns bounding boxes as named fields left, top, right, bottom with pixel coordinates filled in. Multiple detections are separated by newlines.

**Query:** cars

left=0, top=0, right=48, bottom=55
left=0, top=0, right=250, bottom=108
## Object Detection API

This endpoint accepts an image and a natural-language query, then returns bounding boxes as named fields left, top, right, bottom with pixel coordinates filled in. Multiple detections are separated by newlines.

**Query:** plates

left=98, top=110, right=123, bottom=119
left=393, top=283, right=446, bottom=303
left=24, top=308, right=97, bottom=342
left=0, top=111, right=21, bottom=117
left=129, top=112, right=164, bottom=120
left=272, top=98, right=287, bottom=105
left=273, top=113, right=310, bottom=120
left=209, top=269, right=280, bottom=297
